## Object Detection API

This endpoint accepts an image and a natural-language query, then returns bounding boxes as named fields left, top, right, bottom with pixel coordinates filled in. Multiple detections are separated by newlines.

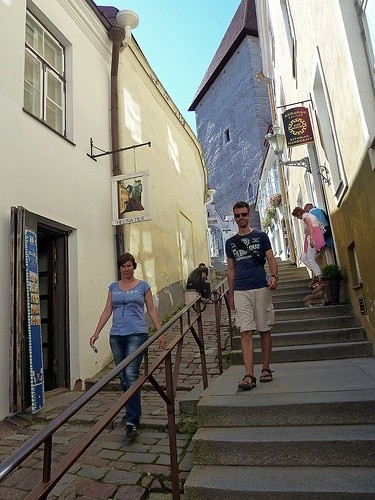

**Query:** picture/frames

left=110, top=169, right=152, bottom=227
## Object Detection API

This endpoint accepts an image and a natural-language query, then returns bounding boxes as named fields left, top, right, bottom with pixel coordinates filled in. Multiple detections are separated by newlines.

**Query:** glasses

left=234, top=213, right=250, bottom=218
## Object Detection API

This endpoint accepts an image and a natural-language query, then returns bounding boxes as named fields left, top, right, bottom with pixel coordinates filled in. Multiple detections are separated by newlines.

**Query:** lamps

left=265, top=121, right=332, bottom=188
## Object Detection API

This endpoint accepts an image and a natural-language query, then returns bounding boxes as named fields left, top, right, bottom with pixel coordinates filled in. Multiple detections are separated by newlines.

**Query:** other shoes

left=206, top=299, right=213, bottom=304
left=310, top=276, right=323, bottom=289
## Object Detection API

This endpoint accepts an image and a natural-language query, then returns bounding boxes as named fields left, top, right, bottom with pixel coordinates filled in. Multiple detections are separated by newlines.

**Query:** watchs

left=272, top=274, right=279, bottom=280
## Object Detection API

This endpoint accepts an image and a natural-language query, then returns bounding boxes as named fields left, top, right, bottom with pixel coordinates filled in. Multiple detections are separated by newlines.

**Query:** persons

left=186, top=263, right=212, bottom=303
left=225, top=201, right=280, bottom=389
left=287, top=203, right=333, bottom=288
left=89, top=253, right=167, bottom=438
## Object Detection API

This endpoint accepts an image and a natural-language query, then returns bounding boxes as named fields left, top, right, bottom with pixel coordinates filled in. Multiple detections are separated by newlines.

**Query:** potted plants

left=319, top=262, right=345, bottom=305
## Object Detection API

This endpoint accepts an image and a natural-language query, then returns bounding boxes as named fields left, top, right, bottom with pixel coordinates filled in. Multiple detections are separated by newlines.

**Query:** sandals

left=260, top=369, right=274, bottom=382
left=238, top=374, right=256, bottom=389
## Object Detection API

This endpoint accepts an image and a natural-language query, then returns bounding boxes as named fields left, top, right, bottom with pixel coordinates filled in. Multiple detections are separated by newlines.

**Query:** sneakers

left=124, top=425, right=141, bottom=439
left=122, top=414, right=126, bottom=422
